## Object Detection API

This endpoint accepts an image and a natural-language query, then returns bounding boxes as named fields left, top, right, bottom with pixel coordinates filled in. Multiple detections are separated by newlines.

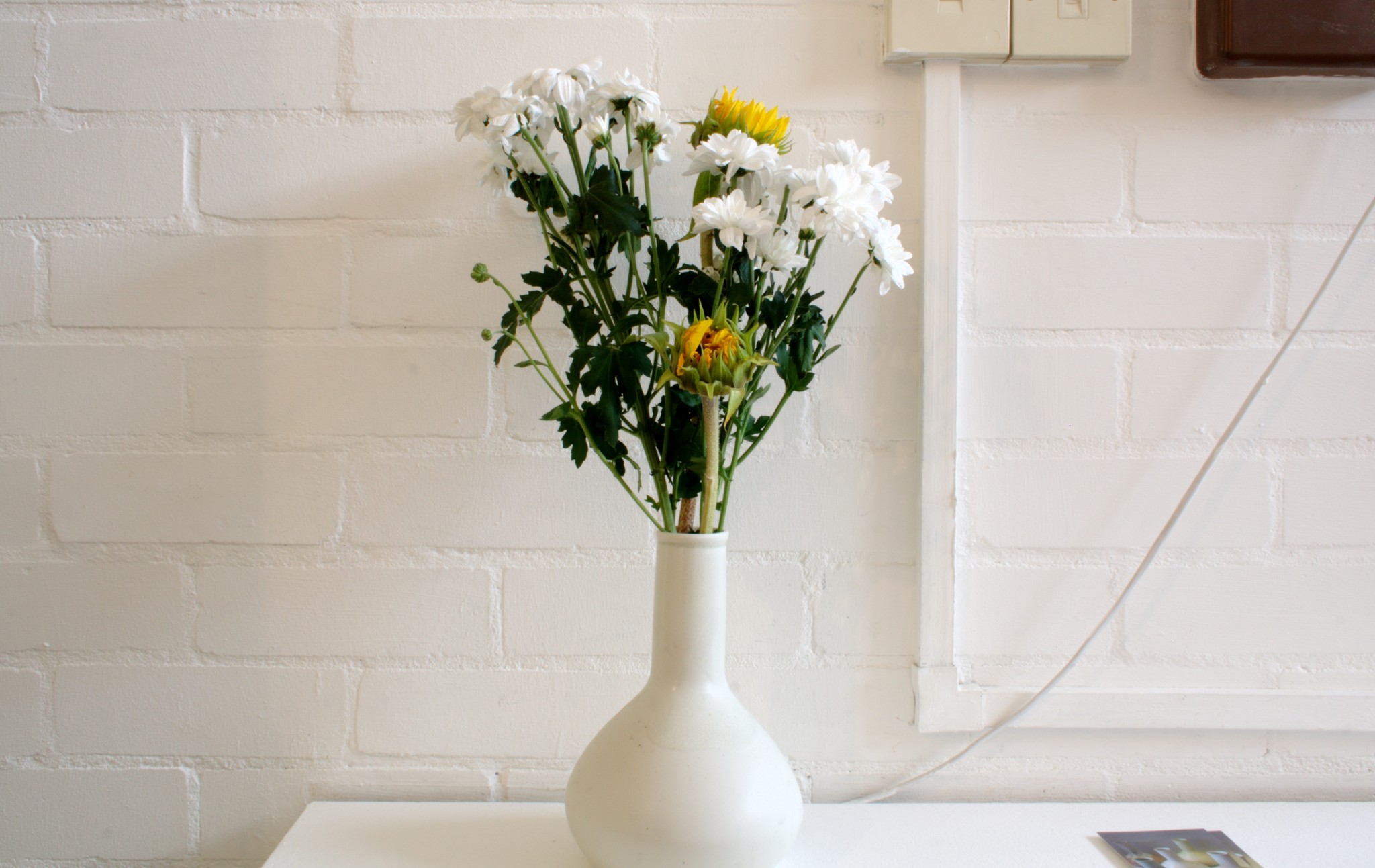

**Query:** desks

left=261, top=801, right=1374, bottom=868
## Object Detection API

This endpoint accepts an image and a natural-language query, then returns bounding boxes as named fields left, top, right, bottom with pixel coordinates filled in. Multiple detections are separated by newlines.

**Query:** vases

left=565, top=535, right=802, bottom=868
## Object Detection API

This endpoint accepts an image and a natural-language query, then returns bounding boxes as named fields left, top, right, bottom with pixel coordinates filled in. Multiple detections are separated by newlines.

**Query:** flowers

left=452, top=59, right=915, bottom=533
